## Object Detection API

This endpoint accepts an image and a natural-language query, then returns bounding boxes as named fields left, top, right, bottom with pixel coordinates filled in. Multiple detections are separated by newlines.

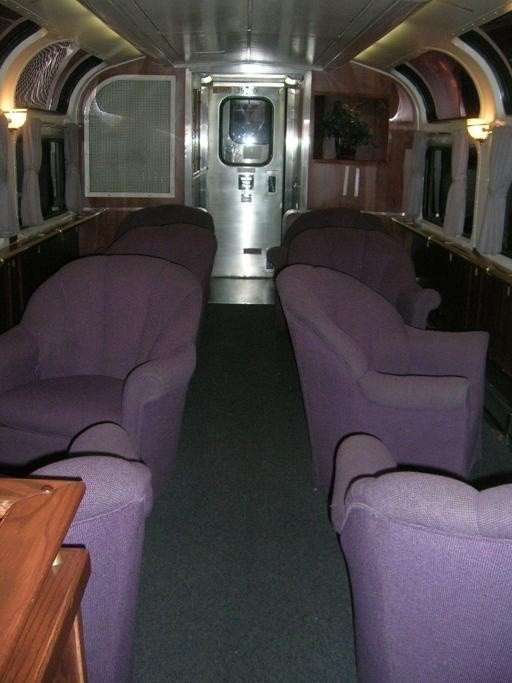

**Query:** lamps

left=466, top=117, right=494, bottom=140
left=2, top=108, right=26, bottom=129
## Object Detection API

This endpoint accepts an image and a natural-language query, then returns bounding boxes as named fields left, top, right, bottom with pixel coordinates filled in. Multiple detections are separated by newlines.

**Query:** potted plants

left=320, top=100, right=375, bottom=160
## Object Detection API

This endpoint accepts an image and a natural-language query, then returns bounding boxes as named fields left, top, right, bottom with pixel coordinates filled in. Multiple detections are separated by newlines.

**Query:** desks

left=1, top=476, right=92, bottom=682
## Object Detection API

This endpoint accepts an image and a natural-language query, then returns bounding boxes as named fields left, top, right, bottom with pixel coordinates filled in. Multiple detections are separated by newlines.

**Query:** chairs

left=26, top=421, right=153, bottom=683
left=95, top=203, right=218, bottom=288
left=274, top=208, right=441, bottom=329
left=1, top=254, right=203, bottom=493
left=329, top=433, right=511, bottom=679
left=276, top=262, right=489, bottom=476
